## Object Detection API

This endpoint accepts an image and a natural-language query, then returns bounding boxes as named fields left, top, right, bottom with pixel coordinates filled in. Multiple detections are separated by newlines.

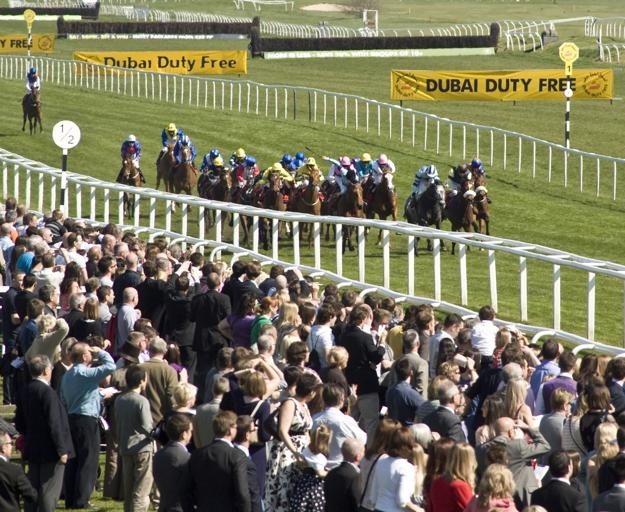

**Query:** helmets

left=245, top=156, right=254, bottom=165
left=457, top=163, right=468, bottom=173
left=339, top=157, right=352, bottom=166
left=471, top=158, right=482, bottom=168
left=30, top=67, right=36, bottom=74
left=282, top=154, right=293, bottom=164
left=236, top=148, right=245, bottom=159
left=181, top=136, right=190, bottom=145
left=272, top=163, right=282, bottom=171
left=167, top=123, right=176, bottom=131
left=426, top=164, right=438, bottom=177
left=376, top=154, right=389, bottom=165
left=304, top=157, right=317, bottom=166
left=127, top=134, right=137, bottom=143
left=212, top=157, right=224, bottom=166
left=295, top=151, right=305, bottom=160
left=360, top=153, right=371, bottom=163
left=210, top=149, right=219, bottom=160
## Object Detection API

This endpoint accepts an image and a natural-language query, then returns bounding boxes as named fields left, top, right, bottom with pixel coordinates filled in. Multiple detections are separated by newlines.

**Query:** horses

left=290, top=168, right=322, bottom=252
left=404, top=177, right=448, bottom=256
left=171, top=144, right=198, bottom=215
left=361, top=167, right=399, bottom=248
left=223, top=180, right=260, bottom=252
left=441, top=177, right=475, bottom=256
left=155, top=144, right=176, bottom=192
left=21, top=85, right=47, bottom=136
left=259, top=169, right=289, bottom=253
left=117, top=153, right=146, bottom=219
left=198, top=169, right=232, bottom=233
left=320, top=178, right=365, bottom=255
left=475, top=172, right=493, bottom=250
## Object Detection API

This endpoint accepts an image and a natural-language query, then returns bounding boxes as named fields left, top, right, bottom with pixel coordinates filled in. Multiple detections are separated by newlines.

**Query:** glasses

left=49, top=234, right=53, bottom=237
left=246, top=427, right=258, bottom=433
left=5, top=441, right=15, bottom=446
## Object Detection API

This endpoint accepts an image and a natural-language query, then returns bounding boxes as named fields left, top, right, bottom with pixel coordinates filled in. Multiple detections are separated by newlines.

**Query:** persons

left=199, top=148, right=226, bottom=196
left=173, top=135, right=197, bottom=167
left=255, top=163, right=294, bottom=207
left=204, top=157, right=227, bottom=186
left=294, top=157, right=320, bottom=180
left=0, top=197, right=625, bottom=512
left=350, top=153, right=373, bottom=183
left=292, top=151, right=308, bottom=168
left=447, top=163, right=472, bottom=198
left=229, top=148, right=246, bottom=172
left=412, top=164, right=439, bottom=203
left=155, top=123, right=184, bottom=165
left=374, top=154, right=396, bottom=176
left=236, top=155, right=260, bottom=188
left=21, top=67, right=40, bottom=106
left=327, top=156, right=356, bottom=192
left=322, top=156, right=356, bottom=193
left=116, top=134, right=146, bottom=183
left=467, top=158, right=485, bottom=177
left=281, top=153, right=296, bottom=171
